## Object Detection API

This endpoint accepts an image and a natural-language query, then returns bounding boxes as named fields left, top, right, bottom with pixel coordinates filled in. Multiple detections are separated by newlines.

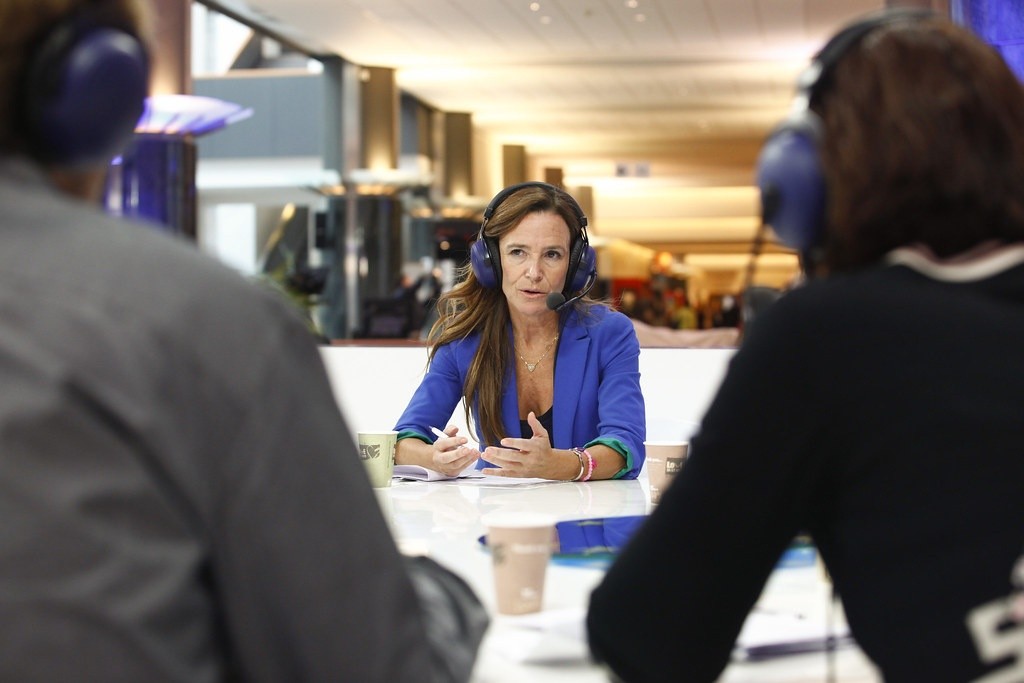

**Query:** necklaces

left=513, top=331, right=559, bottom=373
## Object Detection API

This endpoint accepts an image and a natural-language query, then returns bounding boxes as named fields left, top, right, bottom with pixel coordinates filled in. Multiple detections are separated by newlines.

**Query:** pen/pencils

left=429, top=426, right=465, bottom=449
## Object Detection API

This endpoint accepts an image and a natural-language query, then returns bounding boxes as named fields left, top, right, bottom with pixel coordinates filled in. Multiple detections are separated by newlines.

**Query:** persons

left=0, top=0, right=492, bottom=682
left=616, top=280, right=740, bottom=329
left=584, top=6, right=1023, bottom=682
left=390, top=181, right=647, bottom=483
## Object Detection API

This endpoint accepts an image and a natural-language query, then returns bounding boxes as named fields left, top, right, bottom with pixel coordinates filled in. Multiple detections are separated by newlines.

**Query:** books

left=727, top=547, right=860, bottom=663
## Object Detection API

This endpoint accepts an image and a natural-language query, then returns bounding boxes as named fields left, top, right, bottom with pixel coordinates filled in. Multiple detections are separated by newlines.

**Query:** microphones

left=744, top=201, right=774, bottom=327
left=546, top=271, right=597, bottom=312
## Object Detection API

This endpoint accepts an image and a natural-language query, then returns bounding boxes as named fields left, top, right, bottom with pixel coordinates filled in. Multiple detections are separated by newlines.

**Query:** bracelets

left=562, top=446, right=593, bottom=482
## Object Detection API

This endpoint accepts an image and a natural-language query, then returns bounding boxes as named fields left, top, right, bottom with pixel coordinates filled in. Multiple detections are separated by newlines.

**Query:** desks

left=373, top=456, right=883, bottom=683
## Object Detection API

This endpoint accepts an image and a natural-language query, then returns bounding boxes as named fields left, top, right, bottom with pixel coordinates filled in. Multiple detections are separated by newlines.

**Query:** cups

left=642, top=442, right=689, bottom=503
left=358, top=431, right=400, bottom=487
left=489, top=524, right=553, bottom=615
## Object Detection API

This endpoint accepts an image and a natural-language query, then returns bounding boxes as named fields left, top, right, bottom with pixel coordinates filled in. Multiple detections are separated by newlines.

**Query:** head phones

left=761, top=6, right=944, bottom=251
left=471, top=181, right=597, bottom=292
left=27, top=0, right=153, bottom=173
